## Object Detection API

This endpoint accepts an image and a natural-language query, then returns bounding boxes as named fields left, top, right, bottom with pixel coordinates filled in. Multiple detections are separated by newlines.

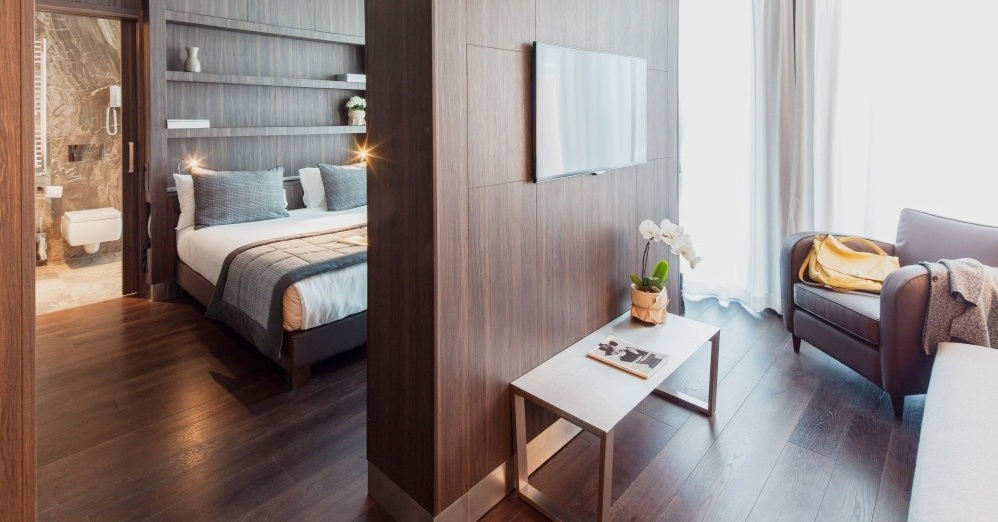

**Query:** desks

left=510, top=308, right=721, bottom=522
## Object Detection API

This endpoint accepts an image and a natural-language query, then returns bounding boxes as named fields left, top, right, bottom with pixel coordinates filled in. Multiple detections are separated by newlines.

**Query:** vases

left=630, top=283, right=670, bottom=325
left=183, top=45, right=202, bottom=73
left=347, top=110, right=366, bottom=126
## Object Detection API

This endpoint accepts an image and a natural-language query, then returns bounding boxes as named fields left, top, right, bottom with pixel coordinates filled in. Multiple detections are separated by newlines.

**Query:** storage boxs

left=166, top=119, right=211, bottom=129
left=336, top=73, right=367, bottom=83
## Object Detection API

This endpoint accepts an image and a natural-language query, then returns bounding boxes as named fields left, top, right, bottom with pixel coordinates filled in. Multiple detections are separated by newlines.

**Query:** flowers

left=629, top=217, right=703, bottom=293
left=345, top=96, right=367, bottom=111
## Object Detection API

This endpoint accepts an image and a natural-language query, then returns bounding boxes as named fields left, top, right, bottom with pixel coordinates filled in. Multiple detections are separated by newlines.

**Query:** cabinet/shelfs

left=165, top=9, right=367, bottom=139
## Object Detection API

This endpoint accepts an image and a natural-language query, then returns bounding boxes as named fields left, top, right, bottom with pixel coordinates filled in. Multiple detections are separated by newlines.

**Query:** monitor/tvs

left=531, top=41, right=648, bottom=184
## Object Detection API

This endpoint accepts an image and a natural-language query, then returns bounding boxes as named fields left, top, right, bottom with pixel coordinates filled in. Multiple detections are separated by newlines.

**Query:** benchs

left=904, top=340, right=998, bottom=522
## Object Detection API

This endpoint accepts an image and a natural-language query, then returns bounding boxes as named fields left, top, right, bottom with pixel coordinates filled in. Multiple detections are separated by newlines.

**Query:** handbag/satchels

left=809, top=233, right=900, bottom=293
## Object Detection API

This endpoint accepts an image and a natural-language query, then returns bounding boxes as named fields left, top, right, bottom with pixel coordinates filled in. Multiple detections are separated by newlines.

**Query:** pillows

left=317, top=161, right=368, bottom=212
left=172, top=172, right=195, bottom=231
left=298, top=166, right=327, bottom=210
left=190, top=165, right=290, bottom=231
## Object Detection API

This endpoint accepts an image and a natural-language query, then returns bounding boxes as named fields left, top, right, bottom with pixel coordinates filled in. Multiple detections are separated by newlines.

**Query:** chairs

left=779, top=207, right=998, bottom=422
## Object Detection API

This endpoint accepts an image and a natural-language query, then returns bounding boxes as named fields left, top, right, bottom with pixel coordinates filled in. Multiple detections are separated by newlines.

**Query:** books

left=588, top=334, right=667, bottom=378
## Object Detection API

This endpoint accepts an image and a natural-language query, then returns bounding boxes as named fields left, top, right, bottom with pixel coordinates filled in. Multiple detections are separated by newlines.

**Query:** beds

left=165, top=174, right=368, bottom=391
